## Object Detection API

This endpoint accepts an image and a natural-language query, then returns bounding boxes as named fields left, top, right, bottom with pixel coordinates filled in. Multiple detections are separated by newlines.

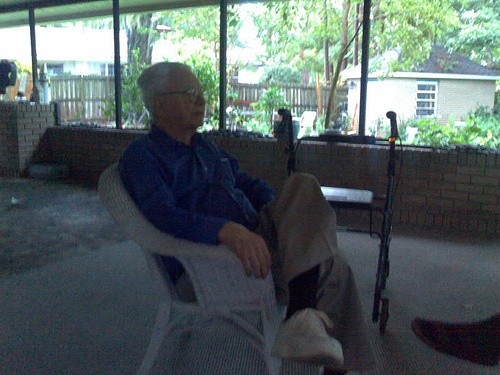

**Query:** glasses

left=160, top=87, right=205, bottom=97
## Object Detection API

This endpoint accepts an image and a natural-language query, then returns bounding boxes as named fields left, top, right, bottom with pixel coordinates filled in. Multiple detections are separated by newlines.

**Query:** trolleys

left=277, top=108, right=401, bottom=334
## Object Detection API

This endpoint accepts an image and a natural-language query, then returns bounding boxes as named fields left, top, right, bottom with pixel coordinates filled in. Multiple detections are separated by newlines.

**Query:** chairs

left=98, top=162, right=281, bottom=375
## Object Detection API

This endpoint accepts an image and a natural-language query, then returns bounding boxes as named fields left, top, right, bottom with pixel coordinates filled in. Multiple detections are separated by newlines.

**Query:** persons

left=117, top=62, right=382, bottom=374
left=412, top=311, right=500, bottom=368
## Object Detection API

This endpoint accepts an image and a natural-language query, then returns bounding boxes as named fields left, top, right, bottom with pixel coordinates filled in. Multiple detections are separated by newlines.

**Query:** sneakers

left=270, top=307, right=344, bottom=369
left=410, top=313, right=500, bottom=366
